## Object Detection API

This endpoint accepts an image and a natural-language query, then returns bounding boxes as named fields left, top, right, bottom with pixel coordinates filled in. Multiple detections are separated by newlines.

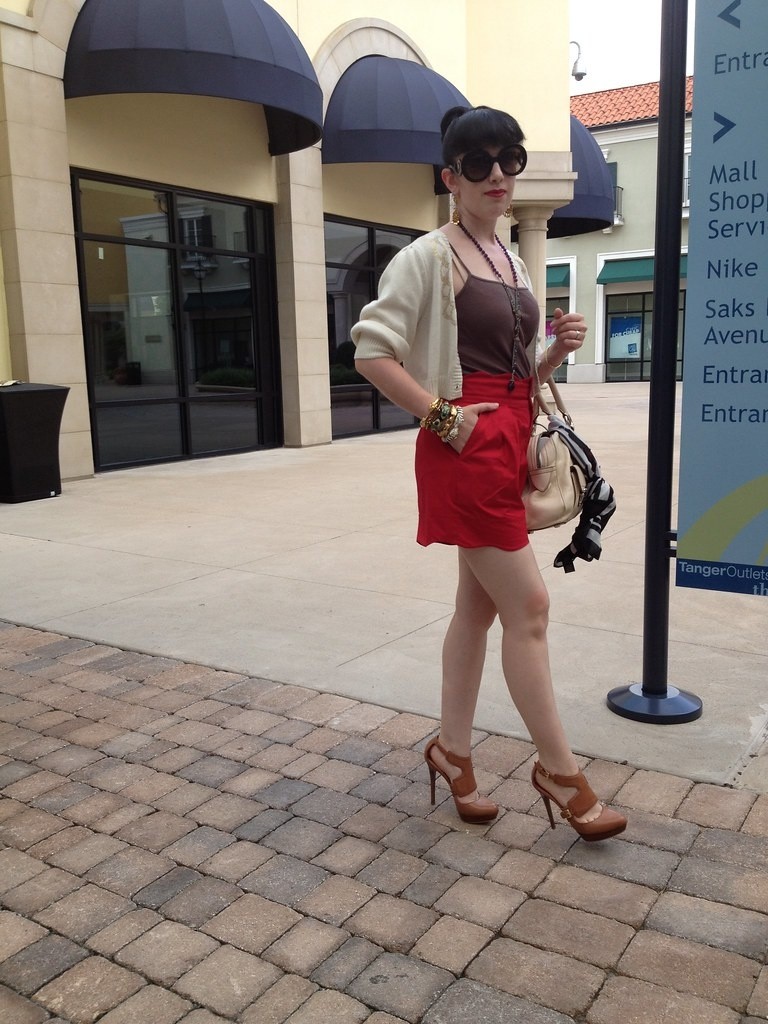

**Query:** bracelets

left=419, top=397, right=458, bottom=438
left=545, top=345, right=563, bottom=369
left=440, top=406, right=464, bottom=442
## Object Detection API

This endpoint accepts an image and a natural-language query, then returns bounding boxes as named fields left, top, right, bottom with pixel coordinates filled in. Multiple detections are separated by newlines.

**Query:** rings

left=576, top=330, right=580, bottom=340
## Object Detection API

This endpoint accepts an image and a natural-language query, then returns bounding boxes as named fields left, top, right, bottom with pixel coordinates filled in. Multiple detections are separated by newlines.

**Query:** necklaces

left=459, top=221, right=521, bottom=391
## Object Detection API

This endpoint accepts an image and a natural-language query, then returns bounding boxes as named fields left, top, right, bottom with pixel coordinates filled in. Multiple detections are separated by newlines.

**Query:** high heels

left=424, top=735, right=498, bottom=824
left=530, top=761, right=626, bottom=843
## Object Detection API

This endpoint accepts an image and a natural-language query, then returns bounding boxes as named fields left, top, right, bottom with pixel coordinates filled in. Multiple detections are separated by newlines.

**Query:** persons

left=349, top=104, right=628, bottom=843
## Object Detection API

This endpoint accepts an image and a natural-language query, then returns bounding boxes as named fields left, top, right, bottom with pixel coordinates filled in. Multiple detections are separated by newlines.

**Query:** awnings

left=546, top=265, right=570, bottom=288
left=63, top=0, right=324, bottom=156
left=511, top=114, right=615, bottom=243
left=183, top=289, right=251, bottom=311
left=321, top=57, right=475, bottom=195
left=596, top=255, right=687, bottom=285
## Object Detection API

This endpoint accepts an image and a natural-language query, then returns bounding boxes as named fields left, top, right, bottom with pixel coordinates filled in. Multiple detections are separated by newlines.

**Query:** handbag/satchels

left=522, top=365, right=601, bottom=535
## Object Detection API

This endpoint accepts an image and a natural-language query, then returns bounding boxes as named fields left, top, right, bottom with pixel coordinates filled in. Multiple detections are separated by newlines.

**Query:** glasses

left=448, top=144, right=527, bottom=183
